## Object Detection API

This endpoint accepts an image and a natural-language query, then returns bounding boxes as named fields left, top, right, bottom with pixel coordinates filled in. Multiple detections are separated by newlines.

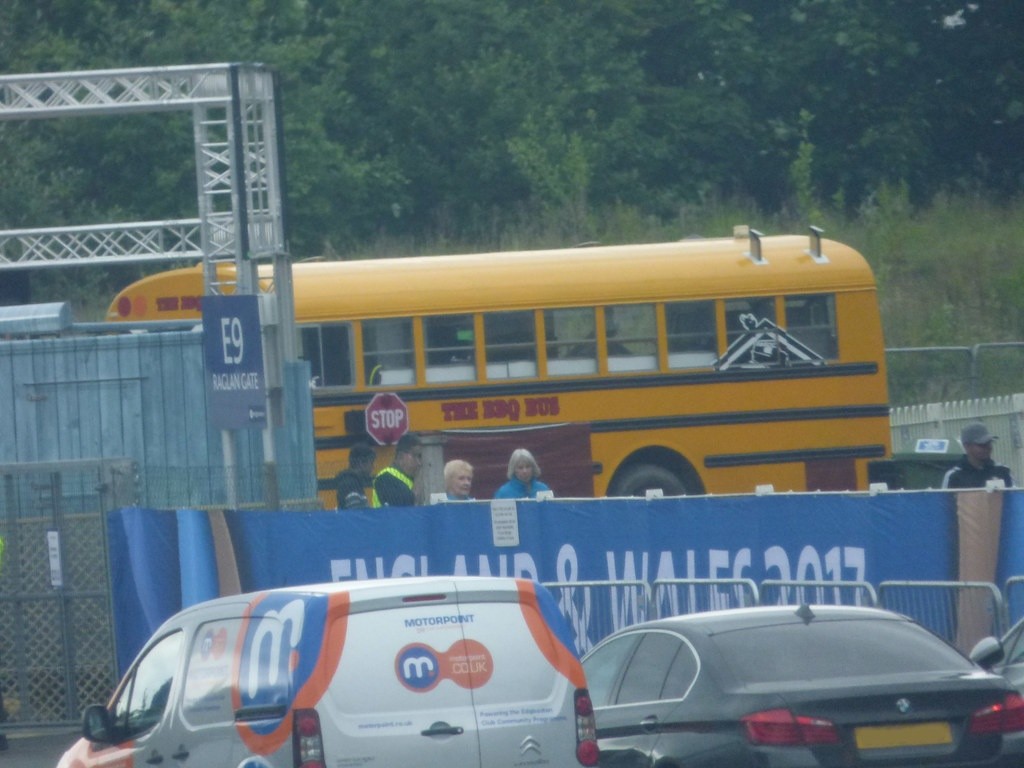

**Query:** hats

left=961, top=423, right=999, bottom=445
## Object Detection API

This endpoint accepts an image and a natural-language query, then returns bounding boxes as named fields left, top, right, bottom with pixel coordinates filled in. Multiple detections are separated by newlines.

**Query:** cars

left=970, top=613, right=1024, bottom=691
left=575, top=602, right=1024, bottom=768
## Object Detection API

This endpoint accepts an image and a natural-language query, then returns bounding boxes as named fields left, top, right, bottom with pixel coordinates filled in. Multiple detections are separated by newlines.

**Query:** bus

left=108, top=225, right=896, bottom=498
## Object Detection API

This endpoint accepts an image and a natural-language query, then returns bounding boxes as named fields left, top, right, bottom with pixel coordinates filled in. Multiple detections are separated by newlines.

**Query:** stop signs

left=364, top=394, right=408, bottom=446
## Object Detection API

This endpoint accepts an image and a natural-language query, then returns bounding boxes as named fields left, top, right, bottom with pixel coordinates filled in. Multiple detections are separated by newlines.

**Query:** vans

left=57, top=575, right=602, bottom=768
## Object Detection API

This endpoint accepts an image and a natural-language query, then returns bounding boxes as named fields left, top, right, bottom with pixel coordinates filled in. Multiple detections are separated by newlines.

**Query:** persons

left=427, top=459, right=477, bottom=506
left=371, top=432, right=422, bottom=507
left=335, top=442, right=376, bottom=509
left=493, top=449, right=554, bottom=500
left=942, top=421, right=1013, bottom=490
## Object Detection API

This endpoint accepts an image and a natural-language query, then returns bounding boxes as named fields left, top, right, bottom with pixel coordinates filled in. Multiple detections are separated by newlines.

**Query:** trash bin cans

left=892, top=452, right=964, bottom=490
